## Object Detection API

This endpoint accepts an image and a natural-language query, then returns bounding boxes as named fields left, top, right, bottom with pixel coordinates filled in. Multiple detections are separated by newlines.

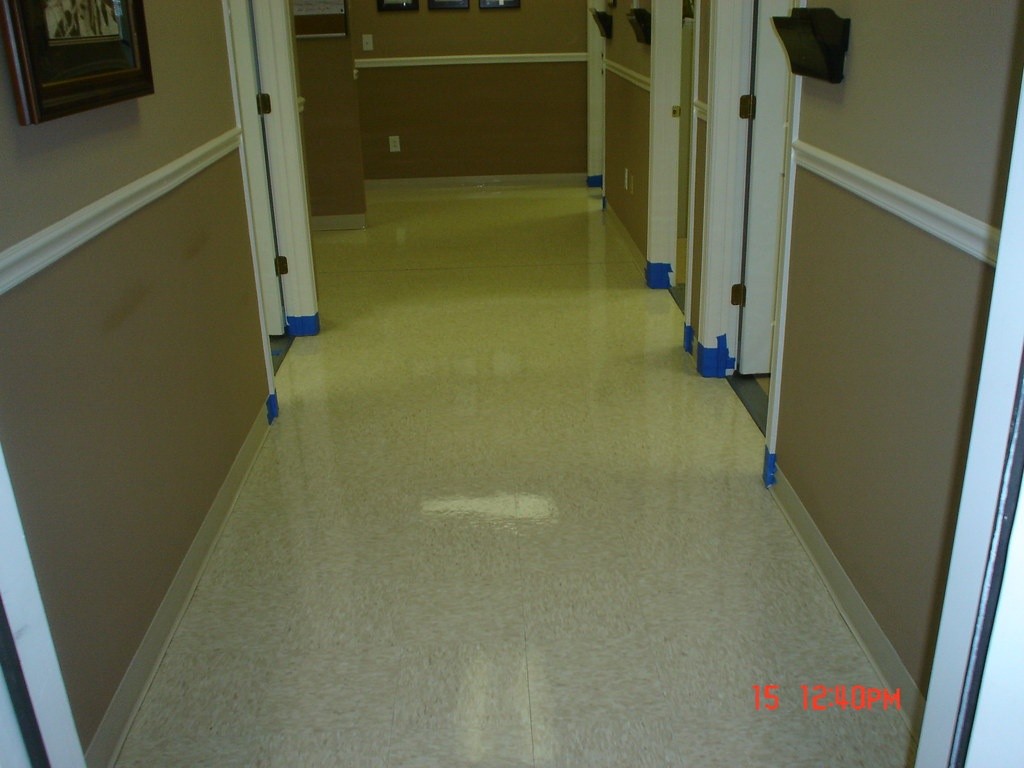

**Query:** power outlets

left=388, top=136, right=400, bottom=153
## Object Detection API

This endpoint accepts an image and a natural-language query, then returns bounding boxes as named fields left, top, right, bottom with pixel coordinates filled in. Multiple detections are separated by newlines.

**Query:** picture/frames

left=0, top=0, right=154, bottom=127
left=377, top=0, right=419, bottom=11
